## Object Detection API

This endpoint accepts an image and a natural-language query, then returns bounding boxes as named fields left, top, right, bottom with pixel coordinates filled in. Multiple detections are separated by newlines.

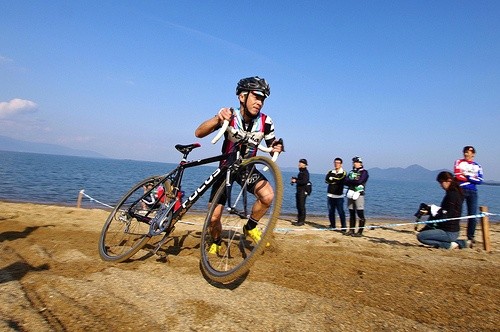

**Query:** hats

left=299, top=159, right=307, bottom=165
left=352, top=157, right=362, bottom=163
left=146, top=182, right=154, bottom=187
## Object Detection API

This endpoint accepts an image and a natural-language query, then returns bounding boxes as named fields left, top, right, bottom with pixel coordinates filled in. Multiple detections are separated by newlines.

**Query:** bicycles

left=97, top=107, right=286, bottom=285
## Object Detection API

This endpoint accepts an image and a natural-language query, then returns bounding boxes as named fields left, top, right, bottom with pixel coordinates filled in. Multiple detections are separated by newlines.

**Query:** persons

left=195, top=76, right=286, bottom=254
left=453, top=146, right=484, bottom=244
left=144, top=180, right=184, bottom=221
left=416, top=171, right=472, bottom=251
left=291, top=159, right=309, bottom=226
left=344, top=156, right=369, bottom=237
left=325, top=158, right=347, bottom=233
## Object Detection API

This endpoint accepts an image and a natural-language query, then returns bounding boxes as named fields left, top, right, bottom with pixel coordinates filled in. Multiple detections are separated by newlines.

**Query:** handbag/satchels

left=304, top=182, right=312, bottom=195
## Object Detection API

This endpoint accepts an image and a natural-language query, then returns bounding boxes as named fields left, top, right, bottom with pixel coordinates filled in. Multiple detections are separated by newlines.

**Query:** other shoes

left=341, top=228, right=346, bottom=233
left=326, top=225, right=336, bottom=231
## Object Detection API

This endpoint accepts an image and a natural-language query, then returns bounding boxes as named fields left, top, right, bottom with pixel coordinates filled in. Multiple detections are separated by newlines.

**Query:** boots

left=345, top=218, right=356, bottom=236
left=351, top=220, right=366, bottom=237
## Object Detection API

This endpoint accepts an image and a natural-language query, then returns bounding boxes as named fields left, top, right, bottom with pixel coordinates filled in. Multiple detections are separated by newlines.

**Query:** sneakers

left=469, top=237, right=476, bottom=243
left=243, top=224, right=271, bottom=246
left=291, top=221, right=304, bottom=226
left=448, top=242, right=459, bottom=249
left=208, top=242, right=226, bottom=255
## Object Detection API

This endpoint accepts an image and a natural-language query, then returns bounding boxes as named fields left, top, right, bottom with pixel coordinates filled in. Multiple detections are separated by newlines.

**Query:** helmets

left=235, top=76, right=271, bottom=98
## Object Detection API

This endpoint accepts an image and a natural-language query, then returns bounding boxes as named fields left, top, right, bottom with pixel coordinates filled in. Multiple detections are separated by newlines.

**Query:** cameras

left=415, top=203, right=429, bottom=219
left=290, top=177, right=296, bottom=186
